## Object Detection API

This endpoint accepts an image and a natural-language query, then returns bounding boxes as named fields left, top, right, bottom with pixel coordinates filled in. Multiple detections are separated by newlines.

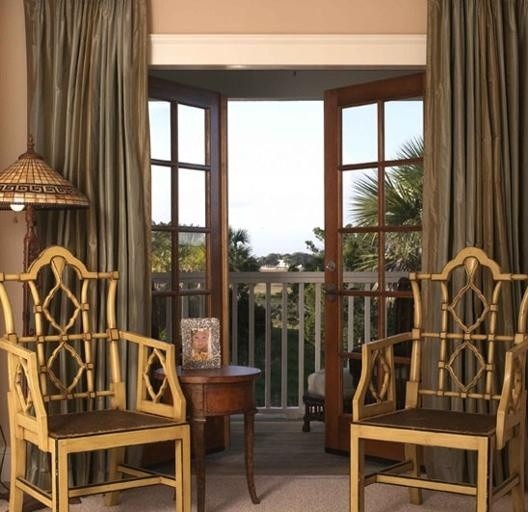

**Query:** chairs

left=343, top=246, right=528, bottom=512
left=0, top=239, right=194, bottom=512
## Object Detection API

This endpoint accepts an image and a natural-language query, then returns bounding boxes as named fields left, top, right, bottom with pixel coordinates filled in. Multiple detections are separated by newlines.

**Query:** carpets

left=0, top=470, right=527, bottom=512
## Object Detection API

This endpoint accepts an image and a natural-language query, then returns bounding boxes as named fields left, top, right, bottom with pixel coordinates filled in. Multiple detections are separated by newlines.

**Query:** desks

left=152, top=362, right=262, bottom=512
left=302, top=395, right=352, bottom=433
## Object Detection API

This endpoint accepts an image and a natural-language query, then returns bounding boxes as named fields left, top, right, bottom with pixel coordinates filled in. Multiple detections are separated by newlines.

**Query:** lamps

left=0, top=132, right=87, bottom=512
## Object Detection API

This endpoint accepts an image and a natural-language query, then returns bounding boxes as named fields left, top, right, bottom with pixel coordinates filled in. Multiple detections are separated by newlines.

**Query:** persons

left=190, top=328, right=209, bottom=360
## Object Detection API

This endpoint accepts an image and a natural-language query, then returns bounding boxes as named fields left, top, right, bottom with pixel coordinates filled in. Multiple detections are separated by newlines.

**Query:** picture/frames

left=178, top=316, right=223, bottom=371
